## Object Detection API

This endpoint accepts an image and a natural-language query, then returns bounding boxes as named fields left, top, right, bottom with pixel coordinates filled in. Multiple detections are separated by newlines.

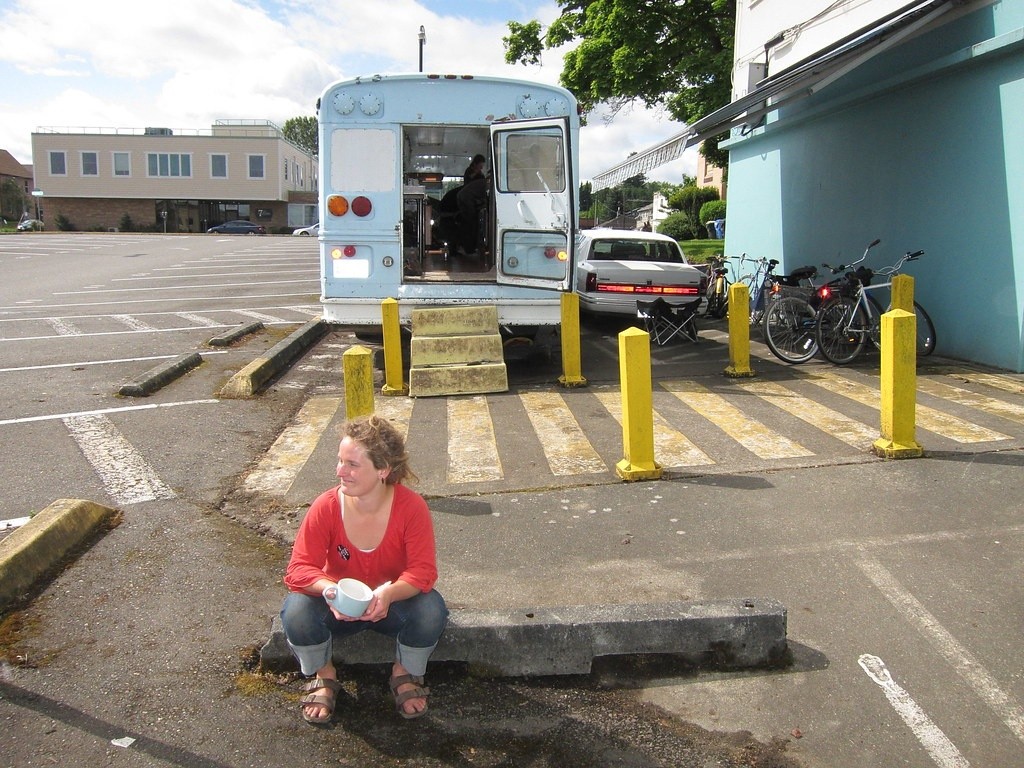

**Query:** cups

left=322, top=578, right=373, bottom=618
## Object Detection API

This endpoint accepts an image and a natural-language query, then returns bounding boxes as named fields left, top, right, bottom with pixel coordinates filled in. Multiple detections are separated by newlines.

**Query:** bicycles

left=815, top=249, right=937, bottom=364
left=703, top=251, right=800, bottom=326
left=762, top=268, right=884, bottom=364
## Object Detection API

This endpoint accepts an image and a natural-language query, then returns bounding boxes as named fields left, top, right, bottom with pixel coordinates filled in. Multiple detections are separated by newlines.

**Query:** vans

left=315, top=72, right=583, bottom=330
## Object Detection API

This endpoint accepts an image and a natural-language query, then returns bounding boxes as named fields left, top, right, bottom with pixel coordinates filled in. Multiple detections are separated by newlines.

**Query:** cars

left=207, top=219, right=266, bottom=234
left=16, top=219, right=45, bottom=232
left=574, top=228, right=708, bottom=316
left=292, top=223, right=319, bottom=237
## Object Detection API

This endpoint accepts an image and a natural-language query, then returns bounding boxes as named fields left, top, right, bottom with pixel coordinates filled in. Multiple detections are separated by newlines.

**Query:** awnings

left=590, top=0, right=939, bottom=192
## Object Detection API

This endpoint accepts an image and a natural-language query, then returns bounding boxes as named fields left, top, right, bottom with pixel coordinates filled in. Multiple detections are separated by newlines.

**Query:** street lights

left=417, top=24, right=428, bottom=73
left=161, top=211, right=168, bottom=233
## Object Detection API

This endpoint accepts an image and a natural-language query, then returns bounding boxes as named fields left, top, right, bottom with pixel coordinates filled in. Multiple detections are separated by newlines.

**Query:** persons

left=432, top=154, right=491, bottom=255
left=280, top=417, right=449, bottom=725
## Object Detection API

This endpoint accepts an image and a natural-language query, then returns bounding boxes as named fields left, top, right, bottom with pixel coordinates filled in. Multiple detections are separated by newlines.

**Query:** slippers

left=302, top=677, right=342, bottom=725
left=389, top=673, right=431, bottom=720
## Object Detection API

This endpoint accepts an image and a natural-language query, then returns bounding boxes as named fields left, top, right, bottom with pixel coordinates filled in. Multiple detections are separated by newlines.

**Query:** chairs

left=633, top=297, right=702, bottom=346
left=610, top=243, right=647, bottom=260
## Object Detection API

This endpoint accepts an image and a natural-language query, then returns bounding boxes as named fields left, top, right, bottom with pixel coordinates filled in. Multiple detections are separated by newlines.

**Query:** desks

left=403, top=194, right=425, bottom=265
